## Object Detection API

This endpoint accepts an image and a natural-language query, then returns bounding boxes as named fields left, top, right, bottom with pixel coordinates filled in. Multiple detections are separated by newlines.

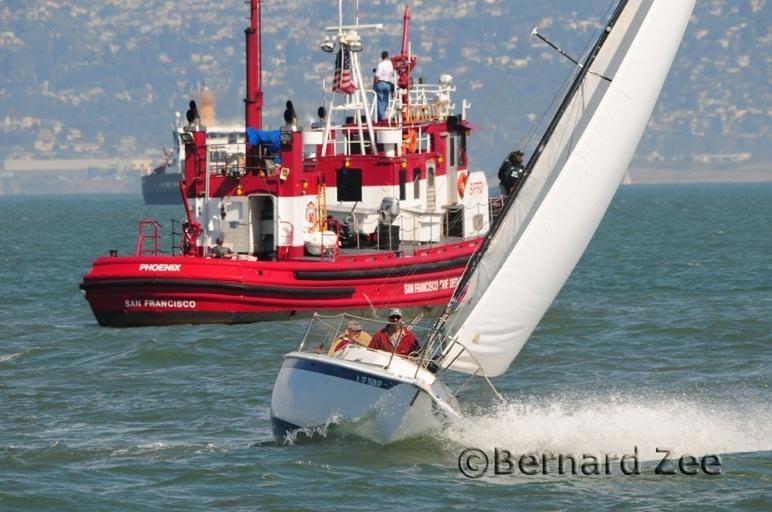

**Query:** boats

left=141, top=112, right=247, bottom=206
left=78, top=1, right=491, bottom=327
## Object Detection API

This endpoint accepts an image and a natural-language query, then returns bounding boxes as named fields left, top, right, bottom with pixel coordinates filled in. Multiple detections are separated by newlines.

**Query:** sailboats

left=269, top=0, right=697, bottom=451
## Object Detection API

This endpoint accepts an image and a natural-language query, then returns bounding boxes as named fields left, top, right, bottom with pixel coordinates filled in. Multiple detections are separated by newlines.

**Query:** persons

left=372, top=51, right=395, bottom=122
left=212, top=237, right=233, bottom=260
left=368, top=307, right=422, bottom=359
left=497, top=150, right=525, bottom=203
left=331, top=320, right=362, bottom=353
left=311, top=107, right=336, bottom=129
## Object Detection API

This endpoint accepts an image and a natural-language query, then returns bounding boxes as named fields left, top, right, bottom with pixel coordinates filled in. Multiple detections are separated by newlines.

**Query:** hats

left=388, top=307, right=402, bottom=319
left=347, top=320, right=362, bottom=332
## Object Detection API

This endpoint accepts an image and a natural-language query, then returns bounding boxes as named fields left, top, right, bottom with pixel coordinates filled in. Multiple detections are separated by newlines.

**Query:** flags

left=332, top=47, right=357, bottom=95
